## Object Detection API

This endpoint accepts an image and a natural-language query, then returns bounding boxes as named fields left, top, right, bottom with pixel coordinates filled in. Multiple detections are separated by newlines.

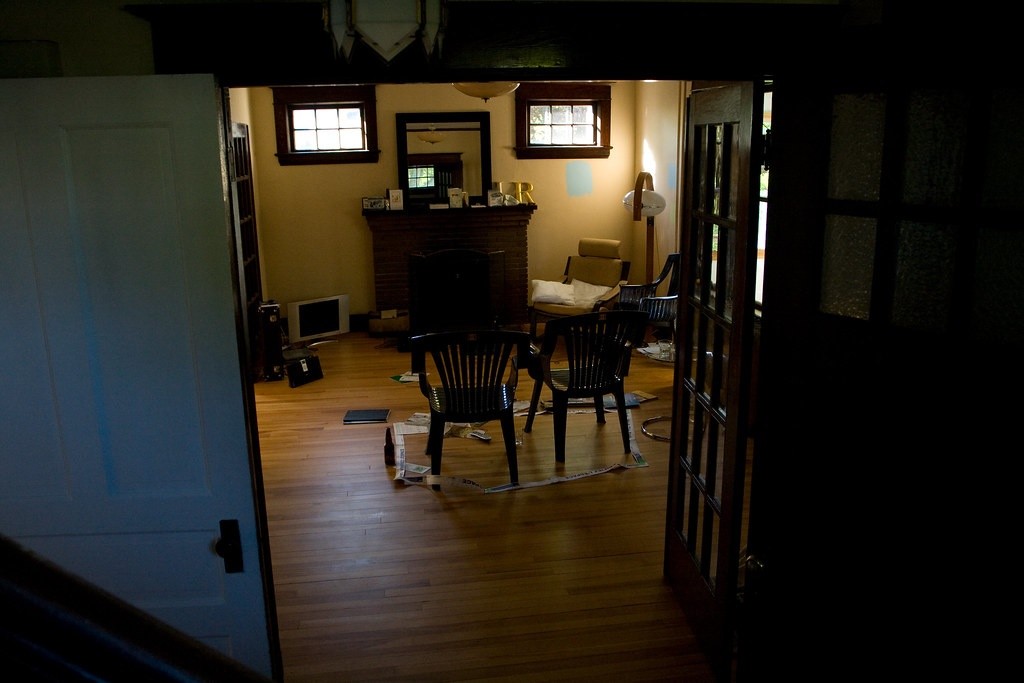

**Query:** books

left=343, top=409, right=391, bottom=423
left=603, top=388, right=658, bottom=410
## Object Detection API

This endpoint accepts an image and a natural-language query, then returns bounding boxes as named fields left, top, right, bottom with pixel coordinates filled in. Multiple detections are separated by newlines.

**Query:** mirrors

left=395, top=113, right=491, bottom=205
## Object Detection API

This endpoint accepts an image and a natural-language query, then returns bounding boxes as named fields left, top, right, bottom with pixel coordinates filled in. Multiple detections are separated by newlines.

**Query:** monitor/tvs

left=287, top=294, right=350, bottom=346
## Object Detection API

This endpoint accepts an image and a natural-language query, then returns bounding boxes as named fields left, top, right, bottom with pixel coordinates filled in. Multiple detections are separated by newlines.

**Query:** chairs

left=618, top=254, right=681, bottom=344
left=410, top=330, right=531, bottom=489
left=531, top=237, right=628, bottom=344
left=530, top=310, right=649, bottom=464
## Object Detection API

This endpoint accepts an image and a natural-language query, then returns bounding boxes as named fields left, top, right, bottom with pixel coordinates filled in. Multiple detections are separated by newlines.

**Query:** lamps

left=622, top=172, right=666, bottom=283
left=418, top=124, right=446, bottom=144
left=450, top=81, right=519, bottom=103
